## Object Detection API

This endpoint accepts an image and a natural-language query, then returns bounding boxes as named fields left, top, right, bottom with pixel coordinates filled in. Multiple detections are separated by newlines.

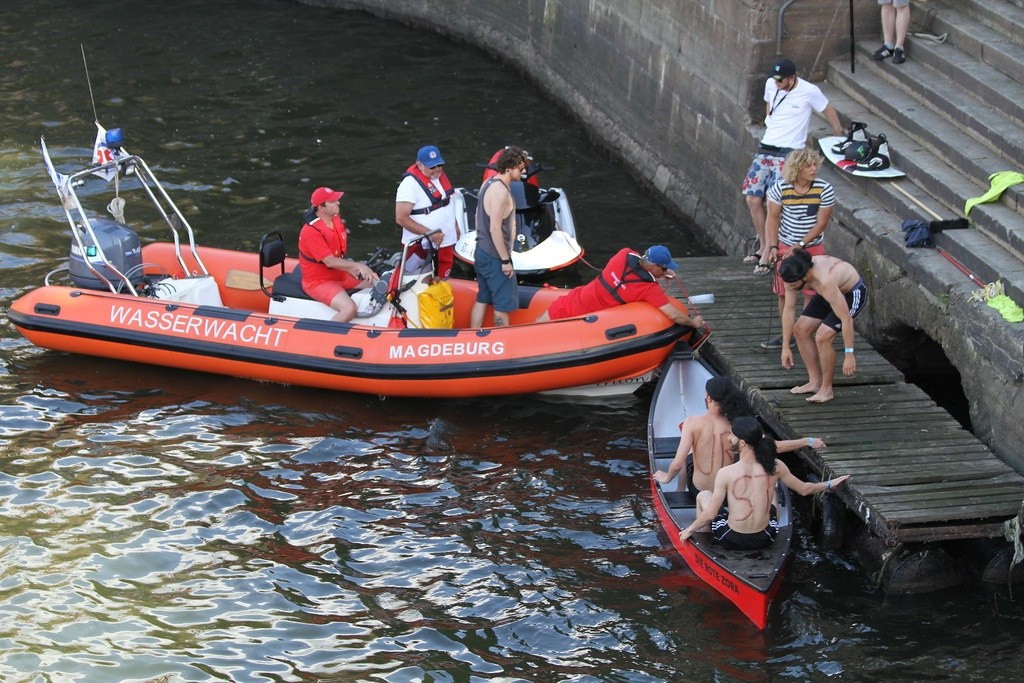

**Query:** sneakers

left=871, top=45, right=894, bottom=61
left=893, top=48, right=905, bottom=64
left=761, top=334, right=797, bottom=349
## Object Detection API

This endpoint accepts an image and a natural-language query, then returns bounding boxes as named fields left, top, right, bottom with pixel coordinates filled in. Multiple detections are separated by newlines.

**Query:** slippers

left=743, top=252, right=761, bottom=263
left=753, top=261, right=774, bottom=276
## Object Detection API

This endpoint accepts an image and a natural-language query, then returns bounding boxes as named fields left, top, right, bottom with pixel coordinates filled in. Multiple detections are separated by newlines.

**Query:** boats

left=644, top=339, right=799, bottom=633
left=7, top=238, right=697, bottom=392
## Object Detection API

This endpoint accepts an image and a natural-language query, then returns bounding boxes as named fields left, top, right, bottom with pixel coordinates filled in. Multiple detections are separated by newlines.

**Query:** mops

left=936, top=247, right=1024, bottom=323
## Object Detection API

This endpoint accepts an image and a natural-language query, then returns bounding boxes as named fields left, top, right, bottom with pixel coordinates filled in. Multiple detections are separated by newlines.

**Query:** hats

left=768, top=60, right=796, bottom=80
left=644, top=245, right=678, bottom=270
left=417, top=145, right=445, bottom=168
left=311, top=186, right=344, bottom=207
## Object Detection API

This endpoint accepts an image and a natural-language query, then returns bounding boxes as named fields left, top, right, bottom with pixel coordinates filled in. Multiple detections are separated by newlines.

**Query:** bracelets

left=845, top=348, right=854, bottom=353
left=501, top=259, right=510, bottom=265
left=809, top=436, right=813, bottom=446
left=768, top=245, right=778, bottom=250
left=824, top=480, right=831, bottom=490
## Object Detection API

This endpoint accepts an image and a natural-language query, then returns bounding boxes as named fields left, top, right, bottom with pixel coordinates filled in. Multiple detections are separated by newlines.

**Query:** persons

left=741, top=57, right=845, bottom=276
left=759, top=148, right=833, bottom=349
left=394, top=144, right=461, bottom=283
left=298, top=187, right=382, bottom=323
left=871, top=0, right=912, bottom=65
left=535, top=244, right=705, bottom=329
left=471, top=146, right=526, bottom=326
left=678, top=416, right=850, bottom=550
left=778, top=246, right=870, bottom=404
left=652, top=376, right=827, bottom=498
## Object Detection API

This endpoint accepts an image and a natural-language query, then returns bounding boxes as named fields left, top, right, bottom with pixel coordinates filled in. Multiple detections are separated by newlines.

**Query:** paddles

left=225, top=269, right=273, bottom=290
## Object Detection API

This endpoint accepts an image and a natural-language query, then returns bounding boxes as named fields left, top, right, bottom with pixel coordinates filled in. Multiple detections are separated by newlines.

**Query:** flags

left=91, top=120, right=135, bottom=182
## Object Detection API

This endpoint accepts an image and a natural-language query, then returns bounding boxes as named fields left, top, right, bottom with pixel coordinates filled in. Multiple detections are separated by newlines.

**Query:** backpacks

left=418, top=280, right=454, bottom=329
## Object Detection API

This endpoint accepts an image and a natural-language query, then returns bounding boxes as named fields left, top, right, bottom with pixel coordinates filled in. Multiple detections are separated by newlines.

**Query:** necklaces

left=769, top=79, right=796, bottom=115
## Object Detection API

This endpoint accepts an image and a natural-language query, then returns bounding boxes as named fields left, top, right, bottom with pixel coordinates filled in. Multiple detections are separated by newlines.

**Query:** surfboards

left=818, top=135, right=906, bottom=178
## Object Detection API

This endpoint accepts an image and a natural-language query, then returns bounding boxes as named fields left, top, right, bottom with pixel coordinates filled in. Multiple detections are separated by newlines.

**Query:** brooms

left=889, top=180, right=969, bottom=234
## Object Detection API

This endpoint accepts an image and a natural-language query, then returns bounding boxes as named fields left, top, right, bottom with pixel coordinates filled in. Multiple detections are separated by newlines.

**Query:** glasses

left=430, top=165, right=442, bottom=169
left=778, top=79, right=782, bottom=83
left=662, top=267, right=667, bottom=271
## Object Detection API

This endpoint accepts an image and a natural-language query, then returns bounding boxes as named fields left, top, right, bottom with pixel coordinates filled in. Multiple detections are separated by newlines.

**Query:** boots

left=831, top=122, right=867, bottom=154
left=857, top=134, right=891, bottom=171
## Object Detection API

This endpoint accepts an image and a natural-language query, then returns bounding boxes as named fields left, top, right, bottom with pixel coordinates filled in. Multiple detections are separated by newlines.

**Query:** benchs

left=663, top=486, right=784, bottom=509
left=653, top=437, right=681, bottom=459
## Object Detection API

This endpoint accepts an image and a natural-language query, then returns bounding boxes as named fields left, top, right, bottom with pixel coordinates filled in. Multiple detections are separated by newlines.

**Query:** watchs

left=798, top=241, right=806, bottom=248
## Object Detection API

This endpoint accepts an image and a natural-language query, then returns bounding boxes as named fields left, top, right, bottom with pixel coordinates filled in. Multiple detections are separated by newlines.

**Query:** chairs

left=258, top=231, right=339, bottom=322
